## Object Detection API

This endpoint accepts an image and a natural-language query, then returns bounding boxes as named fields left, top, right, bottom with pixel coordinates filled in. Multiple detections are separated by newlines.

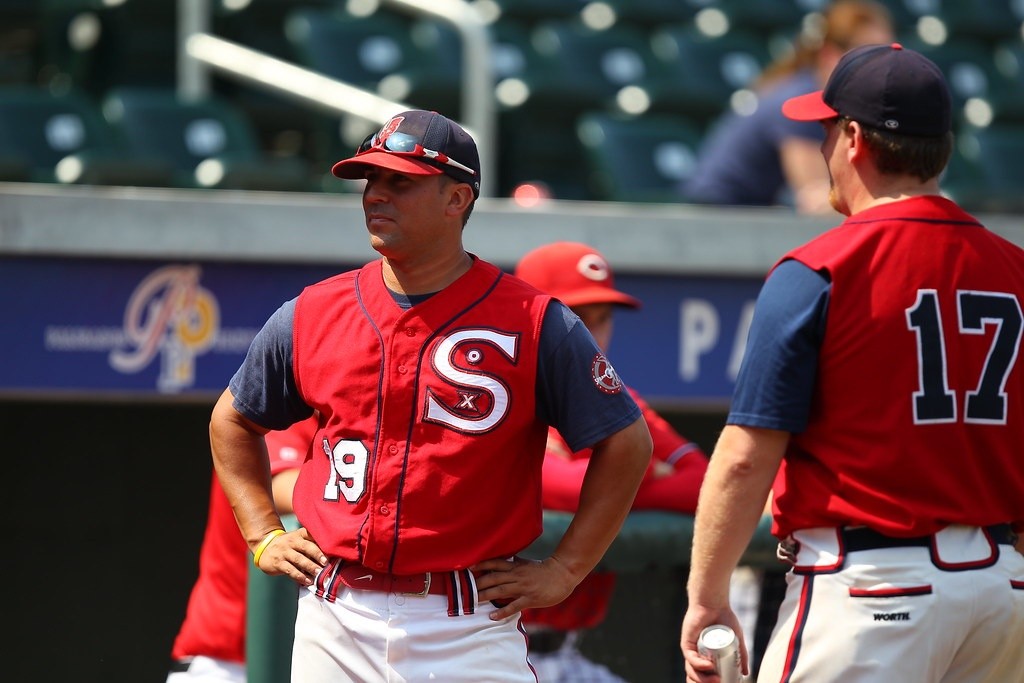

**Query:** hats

left=514, top=242, right=640, bottom=308
left=332, top=111, right=481, bottom=200
left=782, top=44, right=952, bottom=138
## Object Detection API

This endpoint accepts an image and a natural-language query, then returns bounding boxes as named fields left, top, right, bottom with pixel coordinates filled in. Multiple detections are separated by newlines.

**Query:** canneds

left=697, top=625, right=745, bottom=683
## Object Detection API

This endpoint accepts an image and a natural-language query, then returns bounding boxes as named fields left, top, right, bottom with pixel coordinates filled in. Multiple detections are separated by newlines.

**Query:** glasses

left=357, top=134, right=477, bottom=179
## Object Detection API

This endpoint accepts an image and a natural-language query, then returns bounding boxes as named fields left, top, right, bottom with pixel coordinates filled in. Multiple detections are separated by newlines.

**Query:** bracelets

left=252, top=529, right=287, bottom=568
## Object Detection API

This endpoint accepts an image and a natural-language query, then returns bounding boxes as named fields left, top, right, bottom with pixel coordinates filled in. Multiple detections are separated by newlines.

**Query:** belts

left=338, top=560, right=489, bottom=596
left=172, top=658, right=191, bottom=673
left=842, top=524, right=1019, bottom=552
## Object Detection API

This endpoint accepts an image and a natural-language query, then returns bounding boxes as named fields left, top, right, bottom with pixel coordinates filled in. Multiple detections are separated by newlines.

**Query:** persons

left=514, top=242, right=709, bottom=681
left=680, top=3, right=894, bottom=204
left=681, top=44, right=1023, bottom=682
left=167, top=415, right=318, bottom=683
left=210, top=110, right=656, bottom=683
